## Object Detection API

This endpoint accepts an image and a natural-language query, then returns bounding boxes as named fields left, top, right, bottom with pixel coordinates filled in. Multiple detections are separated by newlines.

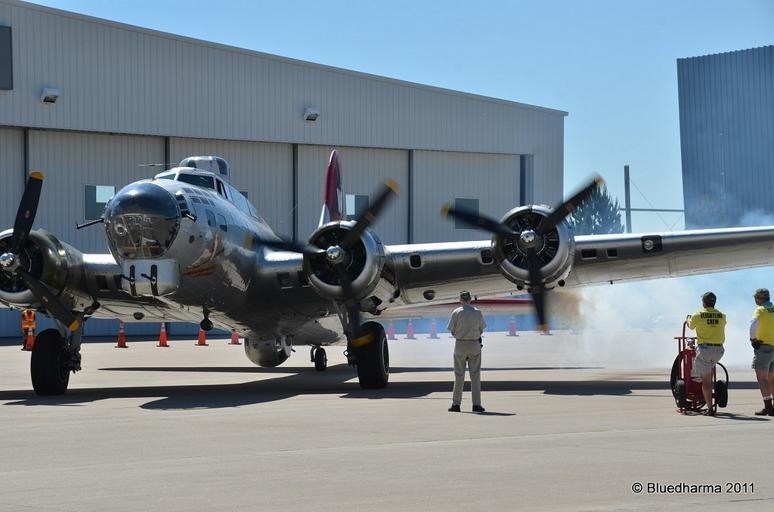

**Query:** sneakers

left=448, top=405, right=460, bottom=412
left=473, top=405, right=485, bottom=412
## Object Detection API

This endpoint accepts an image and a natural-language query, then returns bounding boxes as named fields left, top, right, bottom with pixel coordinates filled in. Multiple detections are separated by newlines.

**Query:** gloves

left=750, top=339, right=760, bottom=350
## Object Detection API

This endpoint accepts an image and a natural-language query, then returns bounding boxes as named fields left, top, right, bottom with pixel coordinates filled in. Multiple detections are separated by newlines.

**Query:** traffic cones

left=196, top=324, right=209, bottom=347
left=116, top=321, right=128, bottom=348
left=228, top=329, right=241, bottom=345
left=22, top=327, right=35, bottom=350
left=428, top=319, right=439, bottom=339
left=506, top=314, right=518, bottom=336
left=405, top=317, right=417, bottom=340
left=156, top=322, right=169, bottom=347
left=386, top=319, right=397, bottom=340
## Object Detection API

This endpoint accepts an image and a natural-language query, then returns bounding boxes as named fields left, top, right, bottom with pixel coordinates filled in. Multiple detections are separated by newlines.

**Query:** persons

left=748, top=287, right=774, bottom=416
left=686, top=291, right=727, bottom=416
left=21, top=310, right=36, bottom=352
left=447, top=290, right=486, bottom=412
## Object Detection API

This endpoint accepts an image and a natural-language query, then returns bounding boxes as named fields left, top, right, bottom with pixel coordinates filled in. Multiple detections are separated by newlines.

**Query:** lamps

left=304, top=108, right=319, bottom=121
left=41, top=88, right=59, bottom=104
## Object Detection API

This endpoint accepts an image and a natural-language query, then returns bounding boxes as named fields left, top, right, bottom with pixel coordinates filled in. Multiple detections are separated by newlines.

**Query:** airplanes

left=4, top=146, right=771, bottom=399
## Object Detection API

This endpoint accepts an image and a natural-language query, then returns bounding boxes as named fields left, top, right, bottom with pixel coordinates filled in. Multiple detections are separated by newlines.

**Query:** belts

left=699, top=344, right=722, bottom=346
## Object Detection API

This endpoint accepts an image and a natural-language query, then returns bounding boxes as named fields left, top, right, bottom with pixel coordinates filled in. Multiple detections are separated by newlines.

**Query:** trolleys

left=671, top=316, right=729, bottom=412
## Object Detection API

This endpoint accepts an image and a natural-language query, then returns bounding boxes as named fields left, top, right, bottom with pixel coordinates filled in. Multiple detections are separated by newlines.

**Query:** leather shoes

left=755, top=407, right=774, bottom=416
